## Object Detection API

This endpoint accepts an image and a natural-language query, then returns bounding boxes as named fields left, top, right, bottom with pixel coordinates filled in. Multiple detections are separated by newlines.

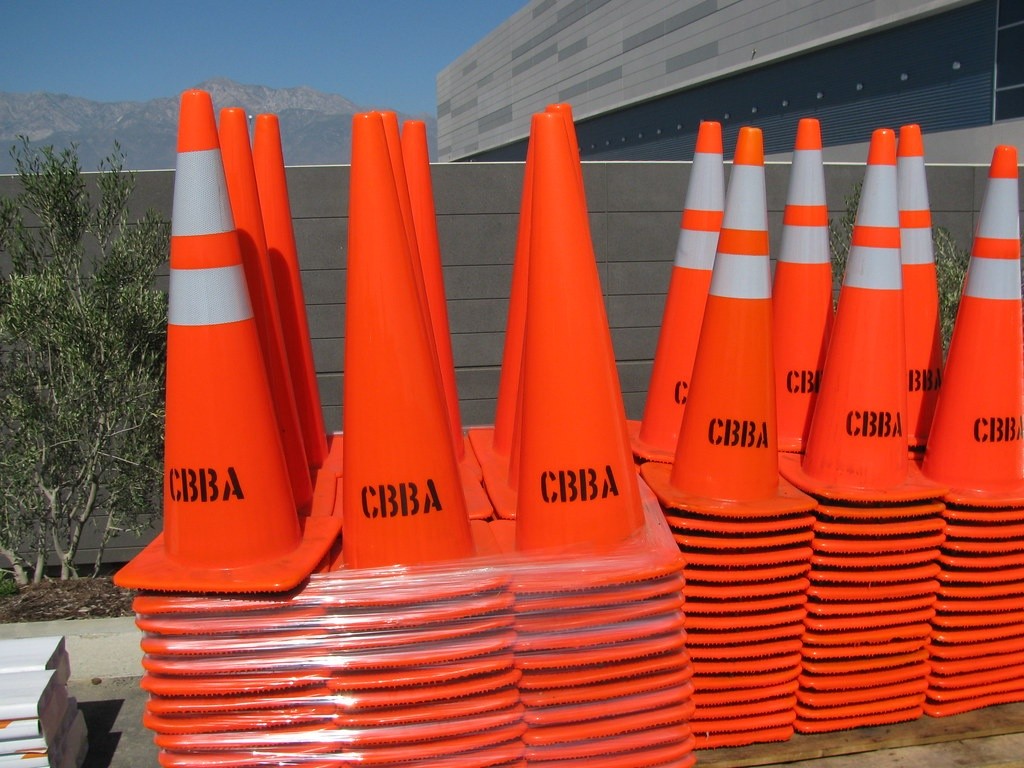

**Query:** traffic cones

left=325, top=112, right=509, bottom=608
left=482, top=102, right=644, bottom=518
left=779, top=129, right=951, bottom=506
left=638, top=127, right=819, bottom=520
left=248, top=110, right=342, bottom=477
left=488, top=110, right=685, bottom=595
left=467, top=101, right=580, bottom=465
left=773, top=118, right=836, bottom=455
left=133, top=149, right=1024, bottom=768
left=397, top=117, right=483, bottom=483
left=896, top=123, right=945, bottom=447
left=111, top=87, right=343, bottom=594
left=216, top=104, right=340, bottom=522
left=622, top=121, right=724, bottom=464
left=918, top=145, right=1024, bottom=511
left=379, top=110, right=499, bottom=521
